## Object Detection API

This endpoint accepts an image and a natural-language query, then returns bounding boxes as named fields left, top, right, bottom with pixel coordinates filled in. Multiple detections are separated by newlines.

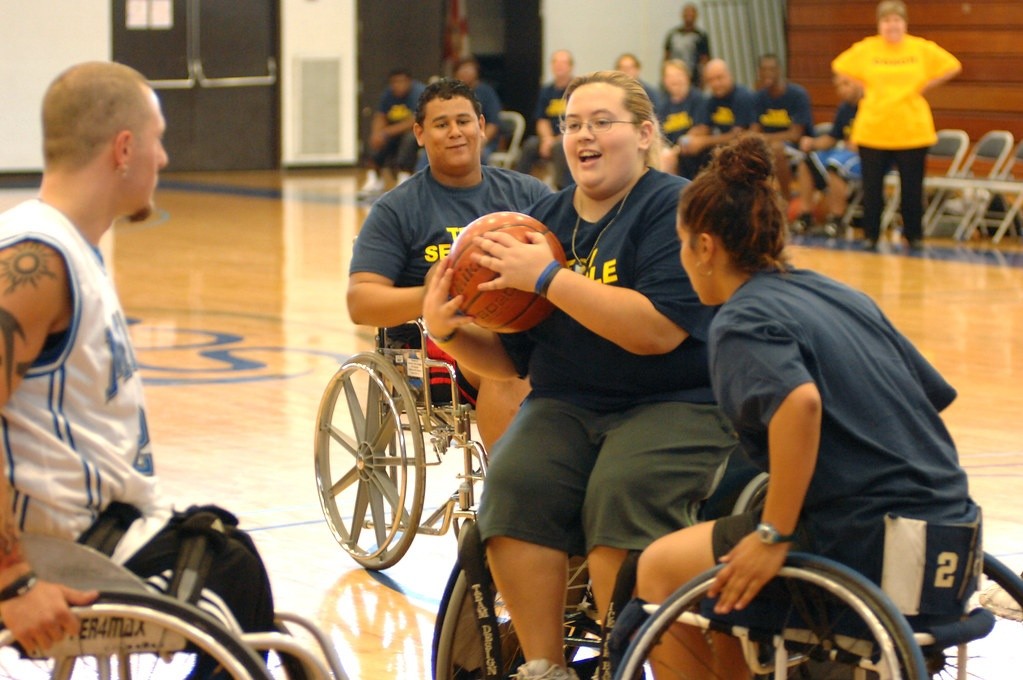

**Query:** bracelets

left=0, top=571, right=37, bottom=600
left=534, top=260, right=562, bottom=298
left=429, top=330, right=457, bottom=344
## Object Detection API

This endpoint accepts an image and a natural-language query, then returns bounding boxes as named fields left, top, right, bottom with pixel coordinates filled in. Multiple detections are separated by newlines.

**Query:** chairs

left=489, top=110, right=526, bottom=170
left=963, top=138, right=1023, bottom=243
left=879, top=129, right=978, bottom=233
left=923, top=130, right=1018, bottom=239
left=841, top=171, right=901, bottom=233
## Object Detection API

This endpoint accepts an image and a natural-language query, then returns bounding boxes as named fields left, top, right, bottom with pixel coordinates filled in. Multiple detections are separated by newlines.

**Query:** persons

left=425, top=72, right=757, bottom=680
left=414, top=56, right=503, bottom=175
left=663, top=4, right=709, bottom=83
left=616, top=52, right=656, bottom=114
left=653, top=52, right=814, bottom=203
left=362, top=69, right=429, bottom=193
left=635, top=135, right=984, bottom=680
left=347, top=77, right=558, bottom=461
left=0, top=61, right=273, bottom=680
left=791, top=74, right=894, bottom=241
left=830, top=0, right=963, bottom=249
left=513, top=48, right=582, bottom=190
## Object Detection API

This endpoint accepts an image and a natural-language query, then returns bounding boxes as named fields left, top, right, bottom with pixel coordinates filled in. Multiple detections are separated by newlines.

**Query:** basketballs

left=446, top=210, right=567, bottom=334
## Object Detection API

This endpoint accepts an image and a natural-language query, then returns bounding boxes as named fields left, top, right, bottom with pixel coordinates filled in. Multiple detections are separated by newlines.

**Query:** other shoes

left=791, top=218, right=810, bottom=234
left=861, top=238, right=877, bottom=249
left=909, top=239, right=925, bottom=251
left=360, top=180, right=382, bottom=196
left=824, top=221, right=838, bottom=238
left=508, top=659, right=579, bottom=680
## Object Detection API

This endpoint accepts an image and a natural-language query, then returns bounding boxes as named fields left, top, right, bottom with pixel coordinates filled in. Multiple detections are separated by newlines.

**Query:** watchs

left=756, top=523, right=796, bottom=545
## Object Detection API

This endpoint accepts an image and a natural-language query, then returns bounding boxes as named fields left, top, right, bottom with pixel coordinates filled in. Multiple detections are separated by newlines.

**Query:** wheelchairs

left=314, top=313, right=493, bottom=573
left=0, top=534, right=349, bottom=680
left=429, top=499, right=1023, bottom=679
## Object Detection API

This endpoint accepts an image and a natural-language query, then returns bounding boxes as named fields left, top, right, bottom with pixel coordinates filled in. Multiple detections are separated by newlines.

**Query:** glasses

left=559, top=117, right=655, bottom=134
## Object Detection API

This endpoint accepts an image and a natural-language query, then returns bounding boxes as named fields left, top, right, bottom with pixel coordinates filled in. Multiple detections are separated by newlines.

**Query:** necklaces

left=571, top=192, right=629, bottom=276
